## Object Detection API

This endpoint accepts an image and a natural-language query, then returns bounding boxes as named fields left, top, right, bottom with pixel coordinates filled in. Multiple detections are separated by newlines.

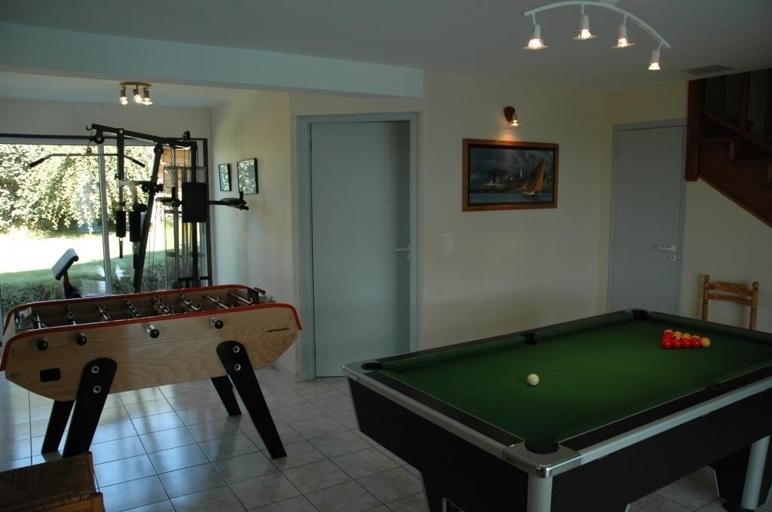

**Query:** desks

left=342, top=306, right=772, bottom=512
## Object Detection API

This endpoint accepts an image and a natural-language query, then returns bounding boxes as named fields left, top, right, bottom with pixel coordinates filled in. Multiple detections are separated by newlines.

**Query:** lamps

left=118, top=82, right=153, bottom=108
left=522, top=0, right=670, bottom=71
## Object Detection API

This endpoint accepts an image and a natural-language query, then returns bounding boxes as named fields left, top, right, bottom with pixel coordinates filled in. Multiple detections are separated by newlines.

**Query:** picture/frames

left=217, top=161, right=231, bottom=193
left=235, top=156, right=258, bottom=195
left=503, top=106, right=520, bottom=129
left=462, top=138, right=559, bottom=212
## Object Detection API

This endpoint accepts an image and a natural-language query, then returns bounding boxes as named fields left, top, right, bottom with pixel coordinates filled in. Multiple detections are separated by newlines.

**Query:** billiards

left=661, top=329, right=711, bottom=349
left=527, top=373, right=539, bottom=385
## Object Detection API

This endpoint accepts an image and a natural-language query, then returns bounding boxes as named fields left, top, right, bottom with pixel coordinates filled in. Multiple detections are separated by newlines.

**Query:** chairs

left=697, top=274, right=759, bottom=330
